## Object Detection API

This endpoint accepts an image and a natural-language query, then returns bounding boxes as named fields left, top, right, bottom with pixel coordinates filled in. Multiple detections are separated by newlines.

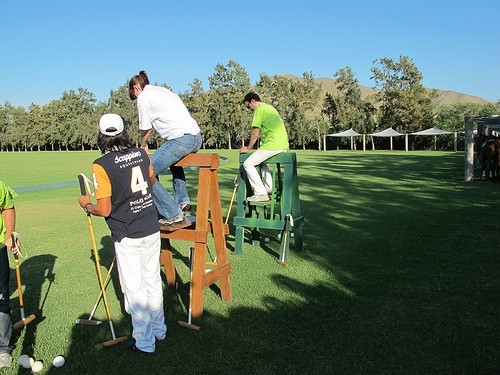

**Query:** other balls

left=290, top=232, right=295, bottom=238
left=264, top=238, right=270, bottom=242
left=277, top=234, right=281, bottom=239
left=52, top=356, right=65, bottom=367
left=17, top=354, right=43, bottom=373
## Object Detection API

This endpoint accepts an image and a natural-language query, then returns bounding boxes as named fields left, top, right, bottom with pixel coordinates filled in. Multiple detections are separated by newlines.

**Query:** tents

left=324, top=125, right=456, bottom=152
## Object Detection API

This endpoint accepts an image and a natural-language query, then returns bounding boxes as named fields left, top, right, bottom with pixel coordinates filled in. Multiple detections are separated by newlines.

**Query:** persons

left=128, top=70, right=202, bottom=226
left=0, top=180, right=19, bottom=368
left=78, top=114, right=167, bottom=354
left=474, top=132, right=500, bottom=183
left=239, top=92, right=289, bottom=202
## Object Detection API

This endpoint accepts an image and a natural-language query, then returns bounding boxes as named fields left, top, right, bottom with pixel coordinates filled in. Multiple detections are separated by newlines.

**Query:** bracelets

left=84, top=203, right=93, bottom=212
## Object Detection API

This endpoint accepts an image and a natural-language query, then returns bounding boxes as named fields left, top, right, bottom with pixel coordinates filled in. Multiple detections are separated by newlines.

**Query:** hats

left=98, top=114, right=124, bottom=137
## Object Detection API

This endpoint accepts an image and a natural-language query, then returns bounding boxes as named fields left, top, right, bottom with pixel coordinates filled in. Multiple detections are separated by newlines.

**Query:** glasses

left=247, top=102, right=250, bottom=109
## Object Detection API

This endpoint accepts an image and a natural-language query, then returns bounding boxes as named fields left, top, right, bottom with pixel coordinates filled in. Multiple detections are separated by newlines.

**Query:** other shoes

left=124, top=342, right=141, bottom=350
left=0, top=353, right=13, bottom=367
left=159, top=215, right=185, bottom=225
left=247, top=194, right=268, bottom=201
left=183, top=204, right=190, bottom=212
